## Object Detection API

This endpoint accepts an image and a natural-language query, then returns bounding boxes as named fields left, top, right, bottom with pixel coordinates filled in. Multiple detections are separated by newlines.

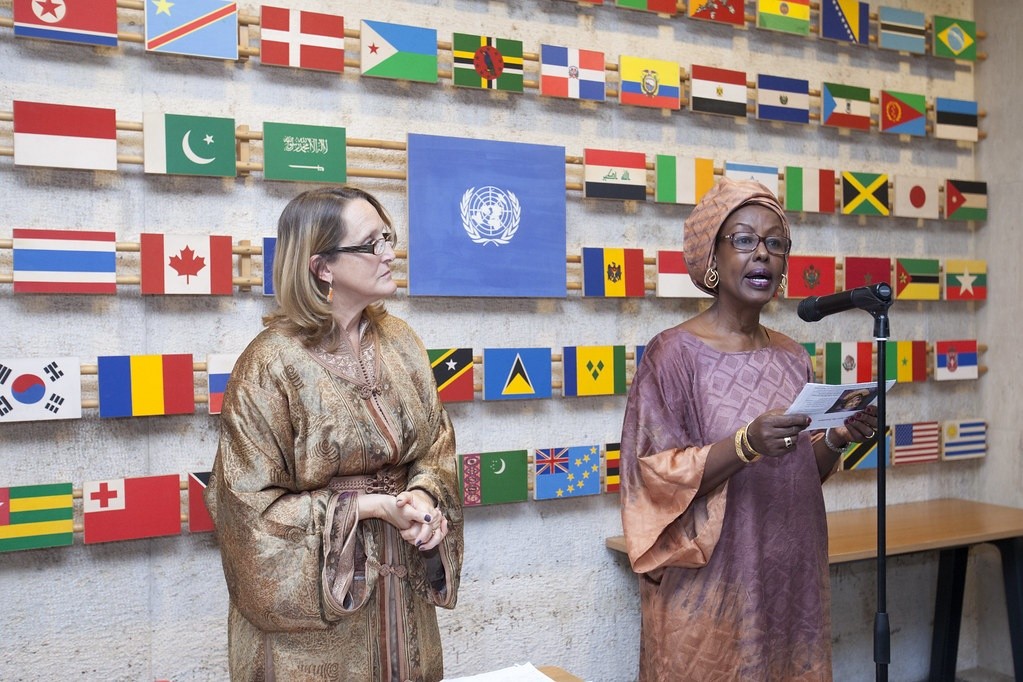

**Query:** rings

left=784, top=435, right=793, bottom=449
left=865, top=431, right=876, bottom=439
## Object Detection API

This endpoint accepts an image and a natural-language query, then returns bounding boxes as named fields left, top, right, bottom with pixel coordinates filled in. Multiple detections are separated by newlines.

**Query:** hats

left=684, top=177, right=791, bottom=293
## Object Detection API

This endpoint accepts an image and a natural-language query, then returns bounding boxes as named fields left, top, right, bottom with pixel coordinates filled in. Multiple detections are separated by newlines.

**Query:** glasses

left=718, top=229, right=797, bottom=260
left=314, top=231, right=397, bottom=256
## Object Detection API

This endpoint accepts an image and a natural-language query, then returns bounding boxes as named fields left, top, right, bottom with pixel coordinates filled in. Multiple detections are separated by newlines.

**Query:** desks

left=606, top=497, right=1023, bottom=682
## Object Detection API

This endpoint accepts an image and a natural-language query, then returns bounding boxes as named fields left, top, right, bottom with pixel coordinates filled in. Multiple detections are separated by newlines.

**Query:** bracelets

left=733, top=426, right=761, bottom=466
left=825, top=427, right=852, bottom=454
left=742, top=420, right=768, bottom=461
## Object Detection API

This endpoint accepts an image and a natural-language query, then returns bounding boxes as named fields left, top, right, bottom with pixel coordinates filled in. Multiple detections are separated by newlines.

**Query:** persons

left=615, top=176, right=886, bottom=681
left=201, top=182, right=467, bottom=681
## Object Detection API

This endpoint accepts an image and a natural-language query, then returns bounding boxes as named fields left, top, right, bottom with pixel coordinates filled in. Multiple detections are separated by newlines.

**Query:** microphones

left=796, top=282, right=894, bottom=322
